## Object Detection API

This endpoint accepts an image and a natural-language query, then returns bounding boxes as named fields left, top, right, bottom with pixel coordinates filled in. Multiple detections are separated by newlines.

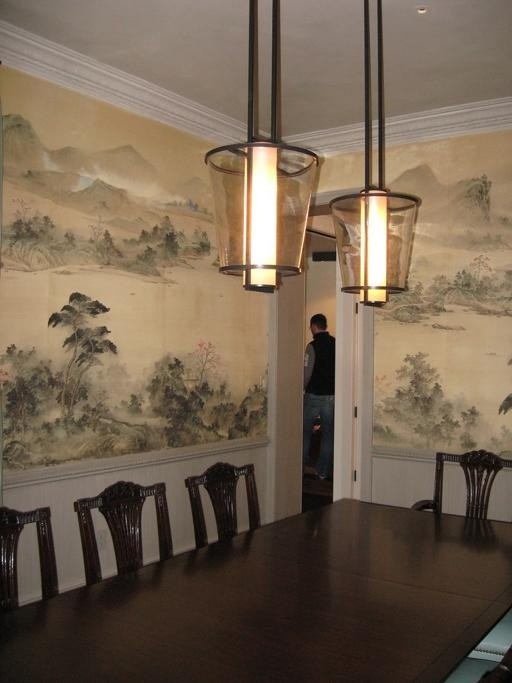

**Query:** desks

left=2, top=490, right=512, bottom=680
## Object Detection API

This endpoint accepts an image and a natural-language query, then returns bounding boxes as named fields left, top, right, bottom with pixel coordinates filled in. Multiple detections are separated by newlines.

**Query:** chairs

left=69, top=477, right=178, bottom=588
left=182, top=457, right=263, bottom=551
left=0, top=503, right=67, bottom=614
left=405, top=447, right=512, bottom=521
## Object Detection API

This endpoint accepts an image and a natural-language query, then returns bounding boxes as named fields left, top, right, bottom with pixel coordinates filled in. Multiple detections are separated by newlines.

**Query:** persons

left=302, top=314, right=335, bottom=481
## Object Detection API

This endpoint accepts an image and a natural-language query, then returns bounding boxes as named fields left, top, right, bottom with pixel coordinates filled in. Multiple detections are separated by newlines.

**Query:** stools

left=476, top=645, right=511, bottom=682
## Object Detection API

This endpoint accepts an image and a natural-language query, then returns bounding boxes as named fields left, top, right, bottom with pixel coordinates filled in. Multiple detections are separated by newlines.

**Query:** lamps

left=325, top=0, right=424, bottom=310
left=202, top=0, right=321, bottom=299
